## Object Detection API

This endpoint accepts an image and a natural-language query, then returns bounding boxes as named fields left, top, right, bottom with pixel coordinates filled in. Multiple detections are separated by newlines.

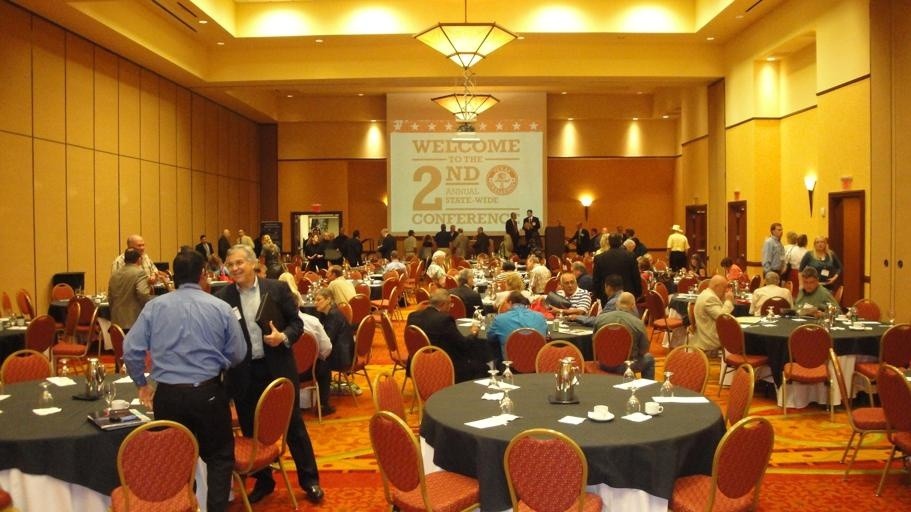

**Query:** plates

left=457, top=318, right=473, bottom=328
left=587, top=412, right=615, bottom=422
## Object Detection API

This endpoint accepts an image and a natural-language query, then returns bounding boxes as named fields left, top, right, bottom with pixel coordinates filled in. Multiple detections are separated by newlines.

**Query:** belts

left=169, top=375, right=223, bottom=391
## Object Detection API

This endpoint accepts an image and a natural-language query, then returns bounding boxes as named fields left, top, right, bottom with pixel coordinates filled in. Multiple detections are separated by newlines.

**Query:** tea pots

left=86, top=356, right=106, bottom=398
left=555, top=358, right=580, bottom=402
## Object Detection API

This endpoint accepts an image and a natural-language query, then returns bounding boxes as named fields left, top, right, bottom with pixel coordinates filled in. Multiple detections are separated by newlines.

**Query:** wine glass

left=499, top=389, right=517, bottom=425
left=626, top=386, right=641, bottom=417
left=622, top=361, right=637, bottom=385
left=37, top=382, right=56, bottom=412
left=304, top=279, right=324, bottom=304
left=488, top=369, right=500, bottom=398
left=661, top=370, right=676, bottom=399
left=473, top=305, right=493, bottom=332
left=501, top=361, right=513, bottom=387
left=654, top=261, right=751, bottom=299
left=59, top=358, right=72, bottom=384
left=102, top=381, right=116, bottom=416
left=761, top=302, right=895, bottom=329
left=548, top=310, right=574, bottom=332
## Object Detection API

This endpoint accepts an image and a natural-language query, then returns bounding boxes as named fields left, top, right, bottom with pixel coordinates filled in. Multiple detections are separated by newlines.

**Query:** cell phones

left=108, top=415, right=122, bottom=425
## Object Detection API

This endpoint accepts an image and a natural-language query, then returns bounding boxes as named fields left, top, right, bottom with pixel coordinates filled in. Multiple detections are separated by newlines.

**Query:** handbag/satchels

left=543, top=291, right=573, bottom=312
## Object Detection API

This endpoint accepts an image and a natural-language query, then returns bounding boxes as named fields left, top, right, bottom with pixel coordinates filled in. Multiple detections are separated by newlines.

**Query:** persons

left=107, top=209, right=844, bottom=512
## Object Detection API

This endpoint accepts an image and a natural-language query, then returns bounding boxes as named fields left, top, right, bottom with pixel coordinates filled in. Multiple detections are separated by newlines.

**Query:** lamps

left=412, top=0, right=518, bottom=132
left=579, top=194, right=595, bottom=224
left=803, top=173, right=818, bottom=217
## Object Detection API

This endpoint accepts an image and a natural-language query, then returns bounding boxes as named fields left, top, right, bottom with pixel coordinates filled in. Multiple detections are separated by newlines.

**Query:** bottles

left=471, top=253, right=557, bottom=299
left=156, top=274, right=173, bottom=285
left=343, top=258, right=388, bottom=284
left=76, top=287, right=110, bottom=304
left=201, top=266, right=226, bottom=281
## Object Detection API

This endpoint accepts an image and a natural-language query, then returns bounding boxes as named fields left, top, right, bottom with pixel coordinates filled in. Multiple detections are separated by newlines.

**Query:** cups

left=645, top=401, right=663, bottom=416
left=120, top=363, right=127, bottom=377
left=594, top=405, right=608, bottom=418
left=110, top=400, right=131, bottom=411
left=0, top=313, right=25, bottom=330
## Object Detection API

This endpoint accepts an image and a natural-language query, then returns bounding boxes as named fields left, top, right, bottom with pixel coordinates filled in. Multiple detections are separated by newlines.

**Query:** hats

left=672, top=224, right=684, bottom=233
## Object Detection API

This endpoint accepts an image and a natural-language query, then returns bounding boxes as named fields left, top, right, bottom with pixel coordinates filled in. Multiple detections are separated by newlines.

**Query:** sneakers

left=321, top=404, right=337, bottom=416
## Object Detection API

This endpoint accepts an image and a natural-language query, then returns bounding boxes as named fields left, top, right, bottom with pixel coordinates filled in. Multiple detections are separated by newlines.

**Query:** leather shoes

left=246, top=477, right=277, bottom=506
left=306, top=485, right=326, bottom=503
